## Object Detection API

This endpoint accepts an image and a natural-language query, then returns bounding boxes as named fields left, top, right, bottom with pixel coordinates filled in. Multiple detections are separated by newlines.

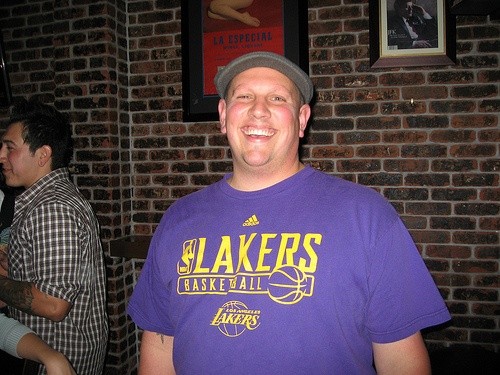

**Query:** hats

left=214, top=51, right=313, bottom=104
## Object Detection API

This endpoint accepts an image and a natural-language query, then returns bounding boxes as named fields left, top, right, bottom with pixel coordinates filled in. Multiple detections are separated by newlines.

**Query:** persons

left=126, top=50, right=453, bottom=375
left=386, top=0, right=439, bottom=51
left=0, top=312, right=80, bottom=375
left=0, top=102, right=109, bottom=375
left=205, top=0, right=261, bottom=28
left=0, top=174, right=26, bottom=310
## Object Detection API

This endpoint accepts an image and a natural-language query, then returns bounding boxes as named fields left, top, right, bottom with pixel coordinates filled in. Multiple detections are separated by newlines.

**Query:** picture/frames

left=181, top=0, right=309, bottom=122
left=369, top=0, right=456, bottom=68
